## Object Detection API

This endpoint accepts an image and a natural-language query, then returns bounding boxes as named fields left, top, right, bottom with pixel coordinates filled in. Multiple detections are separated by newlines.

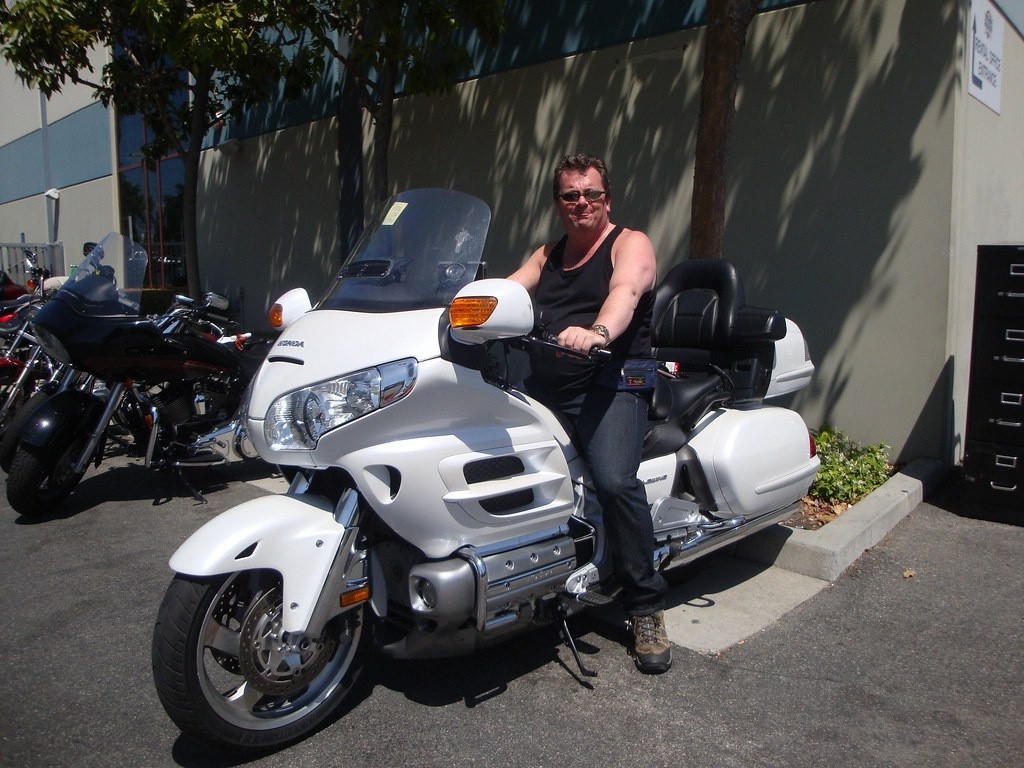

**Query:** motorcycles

left=151, top=188, right=823, bottom=752
left=1, top=232, right=259, bottom=518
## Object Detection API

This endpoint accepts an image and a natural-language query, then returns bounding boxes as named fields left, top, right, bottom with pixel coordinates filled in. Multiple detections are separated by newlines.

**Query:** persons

left=507, top=154, right=673, bottom=672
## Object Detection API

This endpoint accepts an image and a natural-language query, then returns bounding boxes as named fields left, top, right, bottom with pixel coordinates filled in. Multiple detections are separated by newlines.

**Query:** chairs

left=659, top=260, right=739, bottom=403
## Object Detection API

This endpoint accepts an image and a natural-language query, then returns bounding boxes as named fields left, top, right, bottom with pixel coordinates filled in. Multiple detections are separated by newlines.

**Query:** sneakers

left=631, top=611, right=672, bottom=674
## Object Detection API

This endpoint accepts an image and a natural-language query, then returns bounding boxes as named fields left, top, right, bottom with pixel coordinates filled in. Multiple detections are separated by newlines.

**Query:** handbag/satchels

left=598, top=357, right=657, bottom=392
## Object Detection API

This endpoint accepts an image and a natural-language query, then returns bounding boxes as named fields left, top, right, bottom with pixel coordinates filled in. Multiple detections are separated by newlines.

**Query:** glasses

left=558, top=190, right=606, bottom=202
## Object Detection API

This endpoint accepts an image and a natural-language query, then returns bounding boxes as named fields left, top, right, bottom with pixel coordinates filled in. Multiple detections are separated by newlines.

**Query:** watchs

left=589, top=324, right=611, bottom=347
